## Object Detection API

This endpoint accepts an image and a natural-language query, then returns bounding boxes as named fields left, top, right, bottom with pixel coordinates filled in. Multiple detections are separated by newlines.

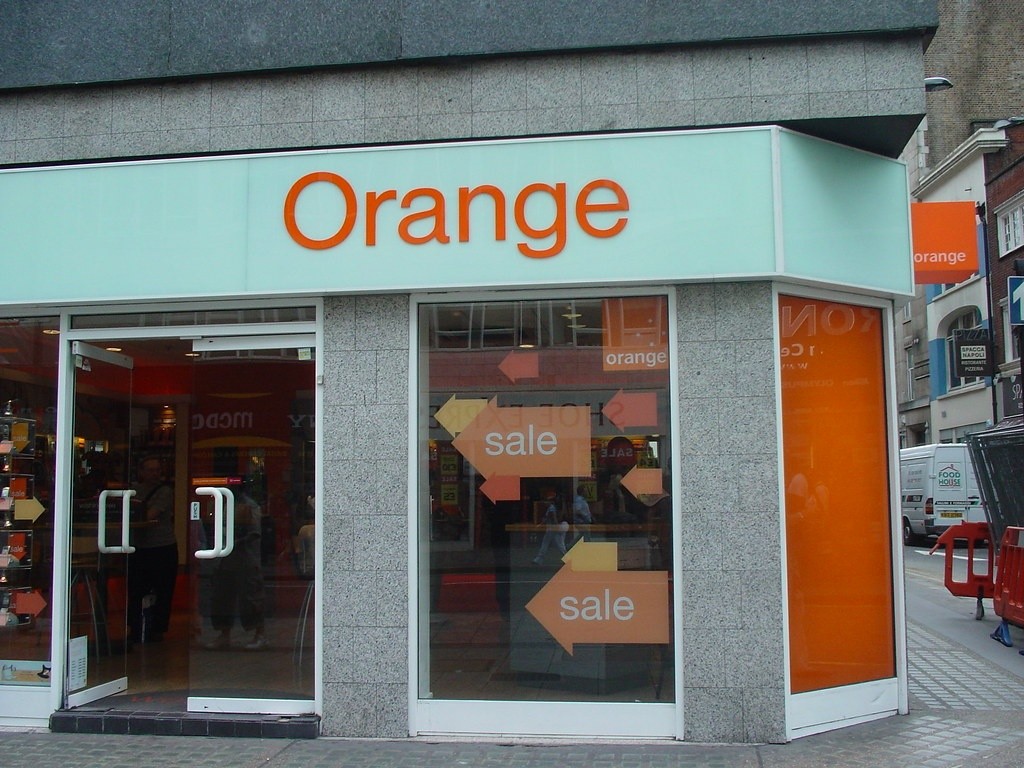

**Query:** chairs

left=70, top=535, right=112, bottom=664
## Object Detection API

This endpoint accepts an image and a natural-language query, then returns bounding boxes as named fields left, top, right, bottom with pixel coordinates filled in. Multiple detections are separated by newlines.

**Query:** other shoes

left=245, top=635, right=269, bottom=650
left=204, top=637, right=231, bottom=650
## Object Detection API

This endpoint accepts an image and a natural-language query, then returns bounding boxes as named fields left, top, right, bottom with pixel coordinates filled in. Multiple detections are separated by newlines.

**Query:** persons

left=204, top=477, right=269, bottom=652
left=560, top=484, right=597, bottom=561
left=532, top=499, right=567, bottom=567
left=97, top=453, right=177, bottom=657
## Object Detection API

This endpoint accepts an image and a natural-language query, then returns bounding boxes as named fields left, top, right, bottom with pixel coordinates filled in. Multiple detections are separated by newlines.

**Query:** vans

left=899, top=442, right=1003, bottom=546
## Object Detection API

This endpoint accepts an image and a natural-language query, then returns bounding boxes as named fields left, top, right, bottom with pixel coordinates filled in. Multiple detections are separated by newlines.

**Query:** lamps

left=925, top=77, right=955, bottom=92
